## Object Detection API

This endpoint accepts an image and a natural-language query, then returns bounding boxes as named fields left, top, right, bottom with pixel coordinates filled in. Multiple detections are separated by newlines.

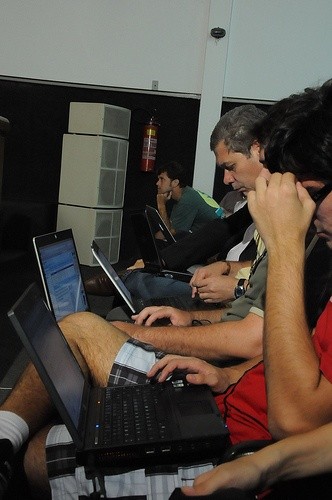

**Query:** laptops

left=142, top=203, right=177, bottom=243
left=134, top=215, right=194, bottom=279
left=6, top=283, right=231, bottom=463
left=86, top=240, right=227, bottom=319
left=31, top=226, right=171, bottom=332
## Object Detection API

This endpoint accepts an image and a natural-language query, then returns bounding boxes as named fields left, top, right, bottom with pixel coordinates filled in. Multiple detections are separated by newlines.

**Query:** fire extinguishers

left=131, top=103, right=161, bottom=174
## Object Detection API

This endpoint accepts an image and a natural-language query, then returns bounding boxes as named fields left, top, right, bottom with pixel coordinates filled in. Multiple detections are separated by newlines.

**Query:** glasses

left=304, top=182, right=332, bottom=206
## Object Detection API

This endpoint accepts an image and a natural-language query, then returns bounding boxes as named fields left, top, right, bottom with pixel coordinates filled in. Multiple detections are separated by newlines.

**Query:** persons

left=0, top=78, right=332, bottom=500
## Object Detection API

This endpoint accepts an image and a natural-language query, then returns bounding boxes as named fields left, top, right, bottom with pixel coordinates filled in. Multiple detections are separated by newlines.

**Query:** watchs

left=235, top=279, right=246, bottom=299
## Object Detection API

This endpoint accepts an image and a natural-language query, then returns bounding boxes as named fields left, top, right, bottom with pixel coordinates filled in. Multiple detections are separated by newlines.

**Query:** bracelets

left=223, top=260, right=231, bottom=275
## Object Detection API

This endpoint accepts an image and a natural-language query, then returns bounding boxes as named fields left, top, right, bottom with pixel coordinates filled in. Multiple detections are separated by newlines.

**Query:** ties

left=238, top=238, right=258, bottom=263
